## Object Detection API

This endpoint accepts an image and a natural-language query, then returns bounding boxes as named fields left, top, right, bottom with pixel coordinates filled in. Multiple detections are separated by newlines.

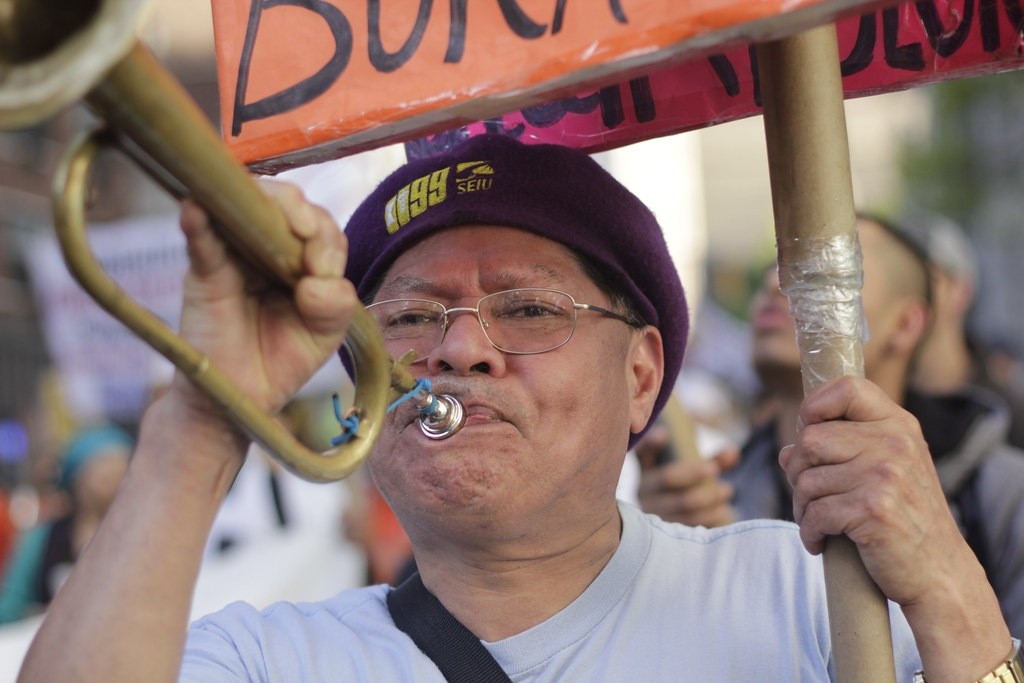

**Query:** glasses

left=364, top=288, right=640, bottom=364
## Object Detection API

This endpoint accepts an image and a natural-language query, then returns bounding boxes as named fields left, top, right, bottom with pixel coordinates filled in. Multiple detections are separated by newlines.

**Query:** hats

left=337, top=133, right=689, bottom=454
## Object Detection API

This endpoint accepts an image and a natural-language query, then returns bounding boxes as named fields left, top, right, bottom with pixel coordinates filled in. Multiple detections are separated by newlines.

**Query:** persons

left=0, top=264, right=423, bottom=683
left=15, top=129, right=1024, bottom=683
left=614, top=202, right=1024, bottom=673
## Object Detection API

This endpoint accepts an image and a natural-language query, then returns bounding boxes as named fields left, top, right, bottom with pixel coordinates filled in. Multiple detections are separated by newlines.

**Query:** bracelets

left=912, top=635, right=1024, bottom=683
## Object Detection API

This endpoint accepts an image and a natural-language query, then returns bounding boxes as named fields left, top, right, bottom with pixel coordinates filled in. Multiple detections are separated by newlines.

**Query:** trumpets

left=0, top=1, right=469, bottom=488
